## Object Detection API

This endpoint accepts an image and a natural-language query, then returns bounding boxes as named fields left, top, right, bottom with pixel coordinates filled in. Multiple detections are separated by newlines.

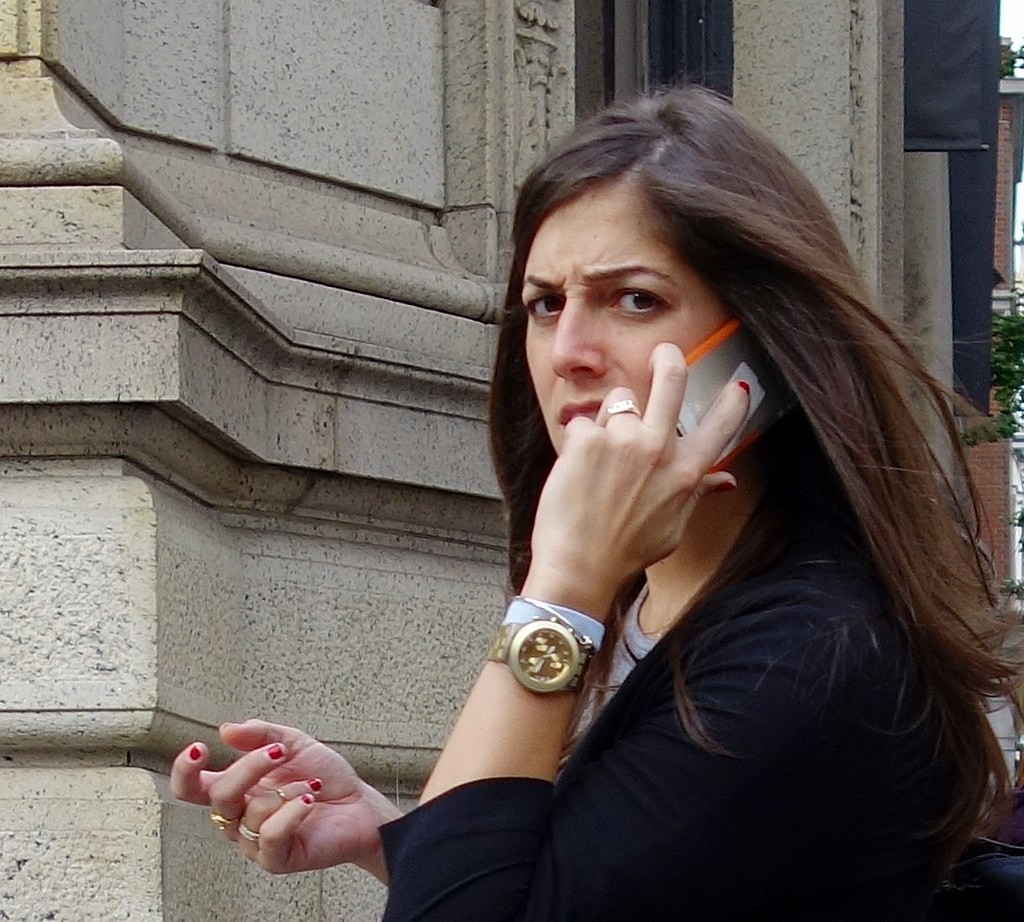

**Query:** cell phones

left=673, top=317, right=797, bottom=471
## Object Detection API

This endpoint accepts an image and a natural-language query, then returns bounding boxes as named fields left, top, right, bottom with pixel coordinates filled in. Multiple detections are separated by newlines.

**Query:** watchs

left=485, top=613, right=599, bottom=696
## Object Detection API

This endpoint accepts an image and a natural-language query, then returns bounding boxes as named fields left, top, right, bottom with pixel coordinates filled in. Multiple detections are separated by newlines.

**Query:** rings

left=237, top=822, right=260, bottom=842
left=207, top=811, right=239, bottom=831
left=275, top=788, right=289, bottom=804
left=600, top=399, right=641, bottom=428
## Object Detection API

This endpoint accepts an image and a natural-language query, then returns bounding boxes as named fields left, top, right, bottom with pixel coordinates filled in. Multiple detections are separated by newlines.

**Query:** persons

left=163, top=88, right=1024, bottom=922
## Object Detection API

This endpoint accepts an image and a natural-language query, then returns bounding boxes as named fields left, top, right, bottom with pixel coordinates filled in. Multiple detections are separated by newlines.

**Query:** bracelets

left=498, top=593, right=606, bottom=653
